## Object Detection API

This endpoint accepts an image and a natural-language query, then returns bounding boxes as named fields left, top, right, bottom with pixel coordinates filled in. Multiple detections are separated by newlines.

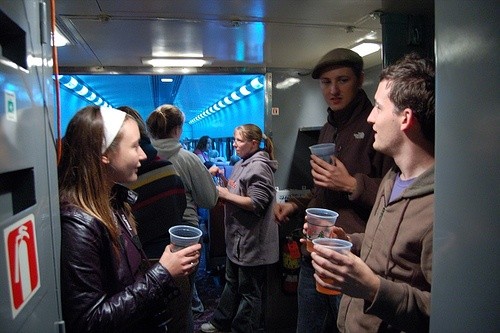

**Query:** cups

left=168, top=224, right=202, bottom=263
left=313, top=238, right=353, bottom=295
left=310, top=143, right=336, bottom=166
left=306, top=208, right=339, bottom=253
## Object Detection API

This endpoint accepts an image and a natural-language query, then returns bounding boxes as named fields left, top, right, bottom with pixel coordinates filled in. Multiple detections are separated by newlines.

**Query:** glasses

left=233, top=139, right=248, bottom=145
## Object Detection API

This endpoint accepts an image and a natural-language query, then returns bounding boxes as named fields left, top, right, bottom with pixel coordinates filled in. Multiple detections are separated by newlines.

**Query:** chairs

left=212, top=165, right=233, bottom=187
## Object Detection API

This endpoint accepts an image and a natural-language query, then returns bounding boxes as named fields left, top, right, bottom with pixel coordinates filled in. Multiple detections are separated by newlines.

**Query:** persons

left=274, top=48, right=394, bottom=333
left=113, top=105, right=278, bottom=333
left=60, top=105, right=202, bottom=333
left=300, top=53, right=435, bottom=333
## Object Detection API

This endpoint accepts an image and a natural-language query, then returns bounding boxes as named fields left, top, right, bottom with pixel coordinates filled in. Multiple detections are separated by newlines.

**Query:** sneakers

left=201, top=322, right=219, bottom=332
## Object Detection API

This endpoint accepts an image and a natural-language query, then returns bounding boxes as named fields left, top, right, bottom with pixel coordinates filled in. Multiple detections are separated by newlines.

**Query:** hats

left=312, top=48, right=363, bottom=79
